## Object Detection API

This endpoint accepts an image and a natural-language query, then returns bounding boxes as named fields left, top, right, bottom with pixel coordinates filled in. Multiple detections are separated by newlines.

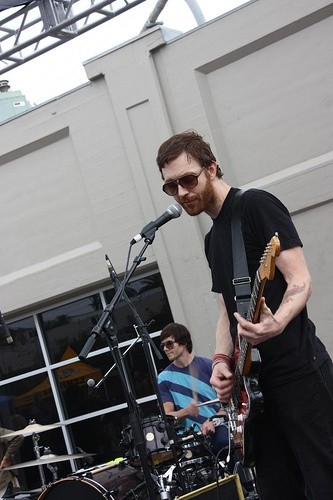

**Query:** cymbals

left=0, top=423, right=64, bottom=438
left=0, top=452, right=97, bottom=471
left=195, top=397, right=221, bottom=407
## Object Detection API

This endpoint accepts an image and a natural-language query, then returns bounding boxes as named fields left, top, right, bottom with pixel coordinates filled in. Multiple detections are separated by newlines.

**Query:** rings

left=252, top=342, right=255, bottom=346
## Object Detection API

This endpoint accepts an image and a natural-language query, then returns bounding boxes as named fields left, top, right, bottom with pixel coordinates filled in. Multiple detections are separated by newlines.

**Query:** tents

left=13, top=344, right=109, bottom=415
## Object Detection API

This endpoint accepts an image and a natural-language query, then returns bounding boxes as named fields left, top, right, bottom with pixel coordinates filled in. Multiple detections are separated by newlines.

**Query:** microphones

left=106, top=257, right=124, bottom=301
left=182, top=422, right=196, bottom=437
left=130, top=203, right=182, bottom=245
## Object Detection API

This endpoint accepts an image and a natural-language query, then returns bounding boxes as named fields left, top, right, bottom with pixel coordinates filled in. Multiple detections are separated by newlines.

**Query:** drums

left=38, top=461, right=149, bottom=500
left=123, top=415, right=184, bottom=467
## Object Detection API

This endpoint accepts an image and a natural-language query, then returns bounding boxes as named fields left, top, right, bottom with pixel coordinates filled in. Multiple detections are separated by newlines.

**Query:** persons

left=156, top=131, right=333, bottom=500
left=156, top=323, right=249, bottom=497
left=0, top=427, right=24, bottom=498
left=9, top=385, right=114, bottom=490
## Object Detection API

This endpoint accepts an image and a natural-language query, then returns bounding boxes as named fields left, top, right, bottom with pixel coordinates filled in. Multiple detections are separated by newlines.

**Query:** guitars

left=229, top=233, right=281, bottom=466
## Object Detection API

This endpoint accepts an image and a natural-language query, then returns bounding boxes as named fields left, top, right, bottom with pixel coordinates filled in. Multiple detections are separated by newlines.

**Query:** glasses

left=161, top=168, right=205, bottom=196
left=159, top=341, right=175, bottom=351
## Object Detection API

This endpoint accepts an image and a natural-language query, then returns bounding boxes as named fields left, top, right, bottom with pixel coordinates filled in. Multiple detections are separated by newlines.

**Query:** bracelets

left=212, top=354, right=231, bottom=369
